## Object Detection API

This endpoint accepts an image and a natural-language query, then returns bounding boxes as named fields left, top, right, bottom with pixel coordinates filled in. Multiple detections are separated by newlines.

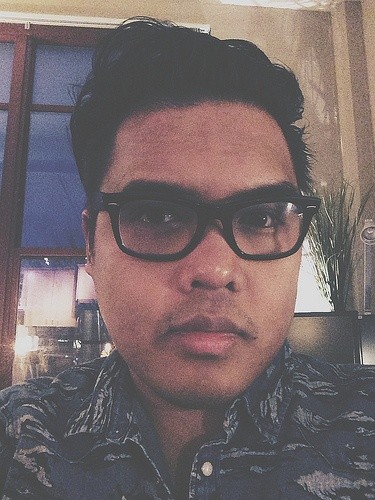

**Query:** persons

left=1, top=15, right=375, bottom=500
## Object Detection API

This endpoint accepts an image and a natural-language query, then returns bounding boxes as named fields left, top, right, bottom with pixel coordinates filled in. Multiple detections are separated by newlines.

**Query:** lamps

left=360, top=218, right=375, bottom=313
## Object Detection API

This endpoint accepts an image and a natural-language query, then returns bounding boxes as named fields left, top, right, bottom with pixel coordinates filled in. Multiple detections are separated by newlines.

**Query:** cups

left=56, top=339, right=73, bottom=356
